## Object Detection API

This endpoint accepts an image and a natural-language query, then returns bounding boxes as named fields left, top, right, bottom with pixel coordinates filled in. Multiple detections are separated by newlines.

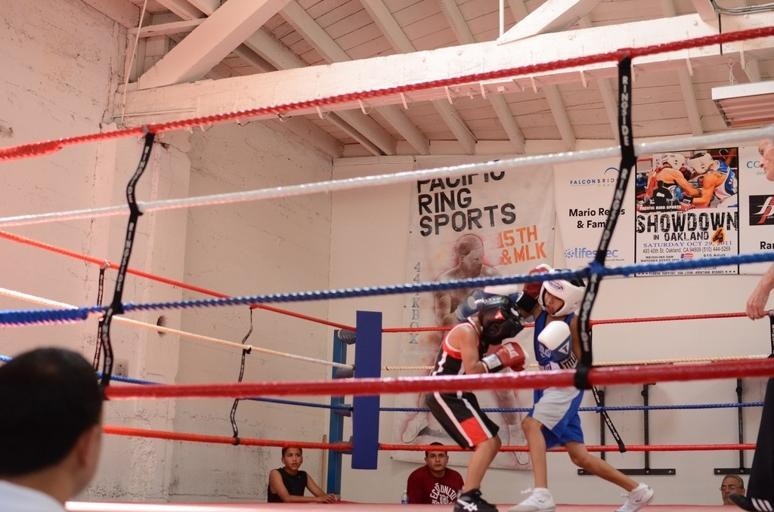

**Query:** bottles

left=400, top=490, right=409, bottom=506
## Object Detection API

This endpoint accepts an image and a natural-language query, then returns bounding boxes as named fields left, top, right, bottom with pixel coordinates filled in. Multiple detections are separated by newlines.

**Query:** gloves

left=454, top=288, right=488, bottom=321
left=522, top=263, right=553, bottom=300
left=484, top=283, right=523, bottom=305
left=537, top=319, right=578, bottom=370
left=482, top=342, right=529, bottom=373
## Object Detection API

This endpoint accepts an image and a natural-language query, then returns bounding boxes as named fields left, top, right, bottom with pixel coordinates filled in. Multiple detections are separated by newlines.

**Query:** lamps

left=711, top=59, right=774, bottom=129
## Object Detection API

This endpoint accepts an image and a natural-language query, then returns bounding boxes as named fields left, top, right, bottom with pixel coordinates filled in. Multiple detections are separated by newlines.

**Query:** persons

left=422, top=264, right=553, bottom=512
left=266, top=445, right=341, bottom=503
left=0, top=345, right=104, bottom=512
left=403, top=441, right=463, bottom=505
left=718, top=473, right=745, bottom=505
left=638, top=148, right=737, bottom=214
left=395, top=233, right=535, bottom=468
left=453, top=267, right=657, bottom=511
left=728, top=124, right=774, bottom=511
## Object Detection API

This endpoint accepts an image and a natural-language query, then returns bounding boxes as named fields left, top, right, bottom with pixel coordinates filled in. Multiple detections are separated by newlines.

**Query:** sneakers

left=453, top=487, right=499, bottom=512
left=401, top=413, right=429, bottom=444
left=507, top=487, right=556, bottom=512
left=509, top=430, right=530, bottom=466
left=729, top=493, right=774, bottom=512
left=615, top=481, right=655, bottom=512
